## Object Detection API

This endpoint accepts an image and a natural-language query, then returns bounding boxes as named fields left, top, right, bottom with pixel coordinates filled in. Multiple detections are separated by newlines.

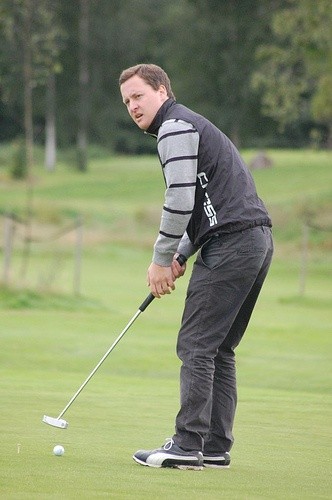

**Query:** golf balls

left=53, top=445, right=65, bottom=456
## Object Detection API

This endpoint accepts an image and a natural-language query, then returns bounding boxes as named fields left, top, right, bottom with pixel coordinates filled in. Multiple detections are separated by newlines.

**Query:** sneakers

left=202, top=451, right=230, bottom=468
left=133, top=439, right=204, bottom=471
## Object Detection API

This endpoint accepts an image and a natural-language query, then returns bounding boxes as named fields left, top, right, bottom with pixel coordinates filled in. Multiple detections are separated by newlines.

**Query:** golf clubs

left=38, top=250, right=179, bottom=429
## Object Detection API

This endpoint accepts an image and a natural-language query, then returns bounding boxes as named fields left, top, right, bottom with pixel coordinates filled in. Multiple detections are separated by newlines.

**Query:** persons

left=119, top=64, right=273, bottom=470
